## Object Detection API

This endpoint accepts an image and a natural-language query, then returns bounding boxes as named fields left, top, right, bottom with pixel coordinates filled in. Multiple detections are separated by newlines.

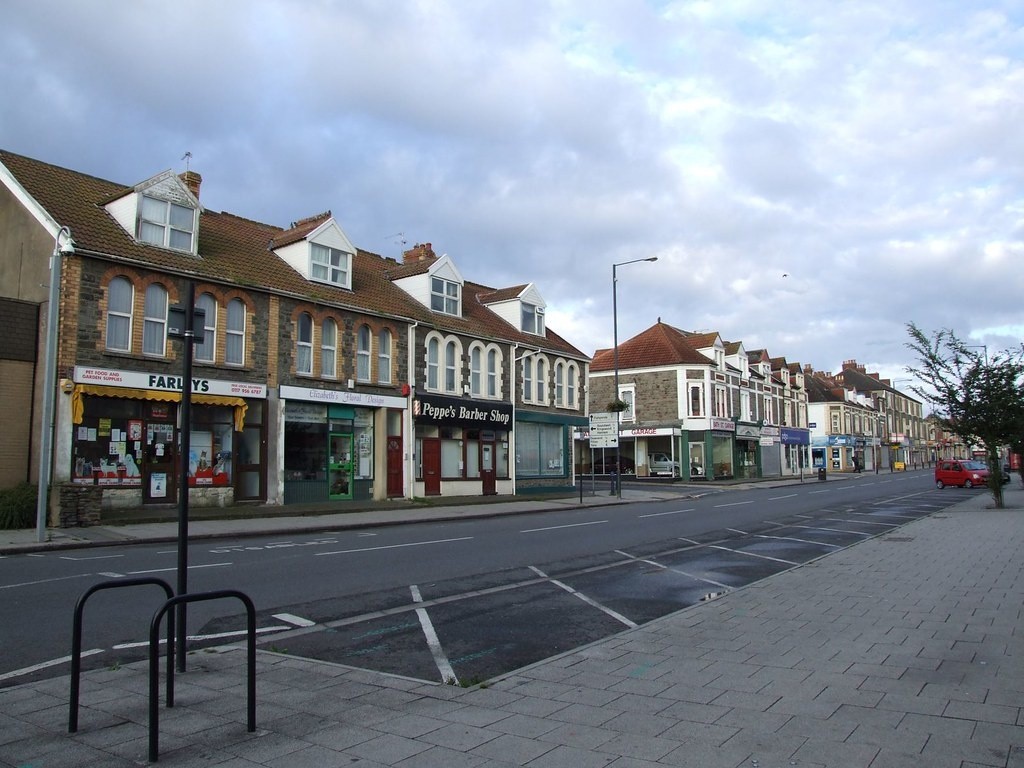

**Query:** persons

left=853, top=455, right=861, bottom=473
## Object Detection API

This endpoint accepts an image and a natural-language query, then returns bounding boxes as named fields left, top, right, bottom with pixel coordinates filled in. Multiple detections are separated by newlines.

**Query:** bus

left=969, top=451, right=987, bottom=465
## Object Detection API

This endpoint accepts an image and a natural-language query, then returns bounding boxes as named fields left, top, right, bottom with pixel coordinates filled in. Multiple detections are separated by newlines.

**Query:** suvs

left=647, top=452, right=703, bottom=476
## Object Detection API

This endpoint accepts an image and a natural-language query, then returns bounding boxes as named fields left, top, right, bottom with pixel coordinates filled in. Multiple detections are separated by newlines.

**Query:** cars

left=591, top=455, right=635, bottom=475
left=935, top=459, right=1011, bottom=489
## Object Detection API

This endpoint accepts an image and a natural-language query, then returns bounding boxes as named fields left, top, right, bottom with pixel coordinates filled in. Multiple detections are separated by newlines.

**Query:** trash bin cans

left=818, top=468, right=826, bottom=480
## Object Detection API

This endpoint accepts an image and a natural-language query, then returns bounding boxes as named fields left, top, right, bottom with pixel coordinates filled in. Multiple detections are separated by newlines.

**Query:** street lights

left=887, top=378, right=913, bottom=474
left=946, top=342, right=990, bottom=380
left=612, top=256, right=660, bottom=495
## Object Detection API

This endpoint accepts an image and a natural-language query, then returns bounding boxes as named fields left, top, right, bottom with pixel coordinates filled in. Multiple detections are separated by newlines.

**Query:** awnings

left=72, top=384, right=247, bottom=432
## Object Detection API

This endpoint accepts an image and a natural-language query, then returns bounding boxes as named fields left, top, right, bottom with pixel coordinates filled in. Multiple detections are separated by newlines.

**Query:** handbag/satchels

left=855, top=465, right=863, bottom=470
